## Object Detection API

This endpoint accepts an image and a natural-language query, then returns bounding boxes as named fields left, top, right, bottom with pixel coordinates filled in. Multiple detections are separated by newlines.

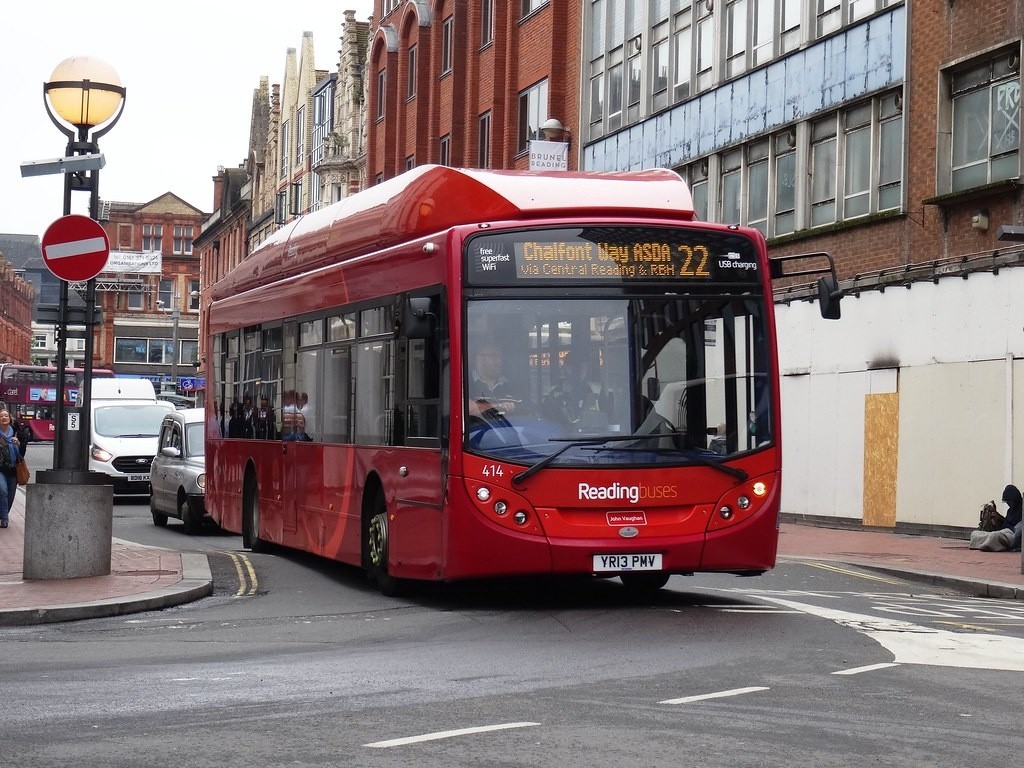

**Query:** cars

left=149, top=408, right=212, bottom=535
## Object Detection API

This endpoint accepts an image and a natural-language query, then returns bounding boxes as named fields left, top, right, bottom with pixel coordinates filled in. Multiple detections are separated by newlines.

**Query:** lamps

left=541, top=119, right=570, bottom=139
left=190, top=291, right=202, bottom=299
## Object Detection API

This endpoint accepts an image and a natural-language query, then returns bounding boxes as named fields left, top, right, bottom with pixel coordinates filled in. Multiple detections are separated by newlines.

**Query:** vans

left=88, top=400, right=177, bottom=499
left=75, top=378, right=157, bottom=408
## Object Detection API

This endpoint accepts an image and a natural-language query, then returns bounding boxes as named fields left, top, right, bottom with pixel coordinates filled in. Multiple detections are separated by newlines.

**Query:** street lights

left=19, top=52, right=125, bottom=585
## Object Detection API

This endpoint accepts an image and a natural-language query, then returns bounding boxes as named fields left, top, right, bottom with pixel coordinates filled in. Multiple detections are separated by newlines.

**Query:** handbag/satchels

left=15, top=456, right=30, bottom=484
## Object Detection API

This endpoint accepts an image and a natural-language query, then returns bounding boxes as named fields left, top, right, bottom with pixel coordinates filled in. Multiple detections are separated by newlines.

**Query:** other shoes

left=0, top=519, right=8, bottom=527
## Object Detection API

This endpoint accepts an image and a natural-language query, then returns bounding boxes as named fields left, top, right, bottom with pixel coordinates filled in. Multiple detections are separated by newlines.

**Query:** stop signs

left=41, top=215, right=111, bottom=282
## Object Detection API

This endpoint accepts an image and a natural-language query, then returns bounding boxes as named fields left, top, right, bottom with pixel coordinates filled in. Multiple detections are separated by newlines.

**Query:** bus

left=0, top=363, right=116, bottom=443
left=202, top=162, right=843, bottom=597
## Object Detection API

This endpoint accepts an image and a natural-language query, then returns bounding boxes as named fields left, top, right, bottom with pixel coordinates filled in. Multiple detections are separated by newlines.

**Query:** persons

left=708, top=423, right=726, bottom=457
left=469, top=342, right=528, bottom=428
left=214, top=391, right=315, bottom=442
left=0, top=400, right=28, bottom=528
left=543, top=351, right=603, bottom=427
left=64, top=390, right=69, bottom=402
left=39, top=389, right=48, bottom=400
left=970, top=485, right=1023, bottom=552
